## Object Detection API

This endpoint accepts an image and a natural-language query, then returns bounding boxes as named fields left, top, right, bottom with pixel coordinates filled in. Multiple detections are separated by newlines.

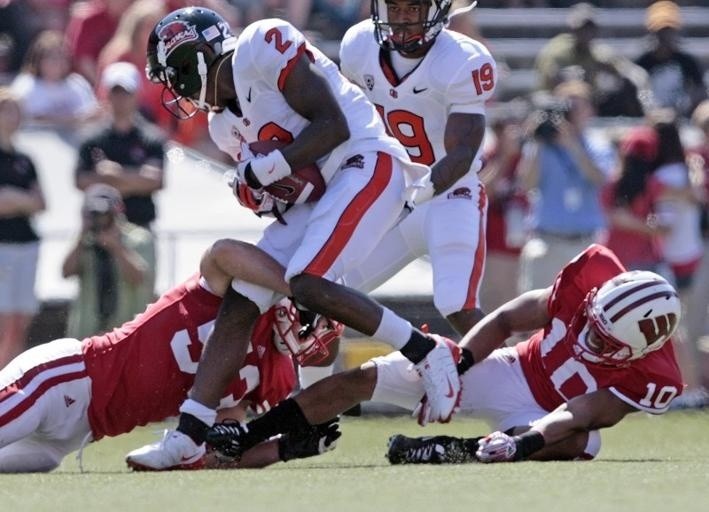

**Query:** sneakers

left=385, top=432, right=460, bottom=466
left=203, top=420, right=249, bottom=464
left=413, top=328, right=464, bottom=427
left=124, top=428, right=208, bottom=473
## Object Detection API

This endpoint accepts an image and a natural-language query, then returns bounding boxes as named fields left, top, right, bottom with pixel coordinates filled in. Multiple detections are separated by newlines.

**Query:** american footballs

left=248, top=140, right=325, bottom=202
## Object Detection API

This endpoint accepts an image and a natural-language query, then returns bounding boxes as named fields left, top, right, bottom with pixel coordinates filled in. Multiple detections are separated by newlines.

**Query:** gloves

left=277, top=417, right=344, bottom=463
left=476, top=430, right=520, bottom=463
left=388, top=201, right=415, bottom=231
left=233, top=146, right=292, bottom=190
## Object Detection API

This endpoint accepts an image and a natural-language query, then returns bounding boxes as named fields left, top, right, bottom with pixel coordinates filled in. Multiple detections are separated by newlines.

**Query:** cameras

left=535, top=103, right=570, bottom=134
left=90, top=197, right=115, bottom=229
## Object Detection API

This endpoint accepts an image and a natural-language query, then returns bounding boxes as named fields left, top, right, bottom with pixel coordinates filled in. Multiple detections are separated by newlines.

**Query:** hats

left=645, top=1, right=687, bottom=35
left=99, top=61, right=142, bottom=99
left=620, top=123, right=660, bottom=164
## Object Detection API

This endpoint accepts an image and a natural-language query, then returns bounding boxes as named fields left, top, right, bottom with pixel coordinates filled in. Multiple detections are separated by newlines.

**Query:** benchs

left=466, top=1, right=709, bottom=138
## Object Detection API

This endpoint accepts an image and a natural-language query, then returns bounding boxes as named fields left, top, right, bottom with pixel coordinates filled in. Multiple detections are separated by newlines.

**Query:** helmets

left=369, top=0, right=452, bottom=53
left=269, top=269, right=347, bottom=363
left=145, top=4, right=234, bottom=107
left=568, top=268, right=684, bottom=370
